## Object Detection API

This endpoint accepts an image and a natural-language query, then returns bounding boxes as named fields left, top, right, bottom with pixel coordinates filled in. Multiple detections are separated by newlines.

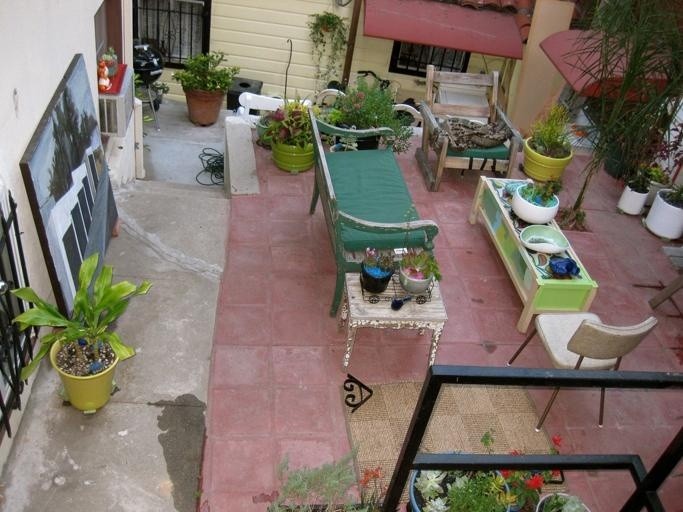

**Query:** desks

left=470, top=176, right=599, bottom=334
left=338, top=273, right=448, bottom=373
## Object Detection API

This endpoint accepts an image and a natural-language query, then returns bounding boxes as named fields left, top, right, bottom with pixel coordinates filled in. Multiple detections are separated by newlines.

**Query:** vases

left=271, top=137, right=315, bottom=175
left=360, top=262, right=395, bottom=293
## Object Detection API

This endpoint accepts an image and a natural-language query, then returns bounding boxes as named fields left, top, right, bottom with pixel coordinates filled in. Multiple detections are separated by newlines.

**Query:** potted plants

left=150, top=82, right=170, bottom=111
left=512, top=97, right=683, bottom=241
left=266, top=439, right=400, bottom=511
left=400, top=250, right=443, bottom=295
left=171, top=47, right=241, bottom=128
left=305, top=0, right=351, bottom=95
left=11, top=253, right=154, bottom=414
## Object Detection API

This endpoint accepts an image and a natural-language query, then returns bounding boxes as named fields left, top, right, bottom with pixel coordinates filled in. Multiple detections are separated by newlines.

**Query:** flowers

left=260, top=73, right=414, bottom=153
left=409, top=428, right=591, bottom=512
left=361, top=246, right=396, bottom=272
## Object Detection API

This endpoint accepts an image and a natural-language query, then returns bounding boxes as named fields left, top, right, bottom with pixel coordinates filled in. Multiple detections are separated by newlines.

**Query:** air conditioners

left=99, top=66, right=135, bottom=137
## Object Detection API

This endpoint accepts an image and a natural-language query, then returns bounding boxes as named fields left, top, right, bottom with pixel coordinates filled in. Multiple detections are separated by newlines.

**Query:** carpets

left=338, top=380, right=570, bottom=503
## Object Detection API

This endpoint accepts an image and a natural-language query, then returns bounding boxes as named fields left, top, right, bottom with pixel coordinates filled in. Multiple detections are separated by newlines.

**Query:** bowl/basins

left=520, top=225, right=570, bottom=254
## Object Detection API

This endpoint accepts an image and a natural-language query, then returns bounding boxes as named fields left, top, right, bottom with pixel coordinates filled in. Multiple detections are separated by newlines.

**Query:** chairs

left=505, top=312, right=659, bottom=433
left=415, top=64, right=522, bottom=190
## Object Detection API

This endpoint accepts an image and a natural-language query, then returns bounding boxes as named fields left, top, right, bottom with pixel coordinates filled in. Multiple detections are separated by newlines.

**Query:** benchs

left=308, top=109, right=439, bottom=319
